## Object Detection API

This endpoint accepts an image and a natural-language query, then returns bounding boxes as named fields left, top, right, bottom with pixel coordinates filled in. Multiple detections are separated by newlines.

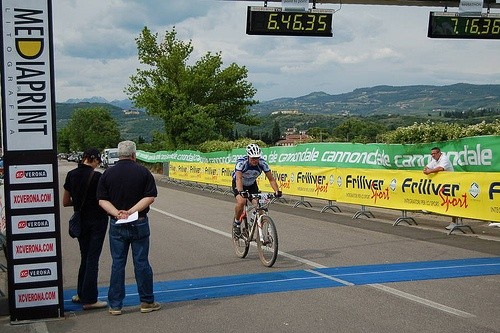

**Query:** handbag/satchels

left=69, top=212, right=82, bottom=238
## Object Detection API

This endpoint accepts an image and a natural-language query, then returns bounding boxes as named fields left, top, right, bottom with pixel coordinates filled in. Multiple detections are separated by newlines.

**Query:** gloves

left=238, top=190, right=250, bottom=198
left=274, top=191, right=282, bottom=197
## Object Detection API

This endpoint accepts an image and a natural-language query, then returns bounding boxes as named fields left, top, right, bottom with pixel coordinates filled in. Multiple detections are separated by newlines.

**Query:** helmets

left=246, top=144, right=261, bottom=157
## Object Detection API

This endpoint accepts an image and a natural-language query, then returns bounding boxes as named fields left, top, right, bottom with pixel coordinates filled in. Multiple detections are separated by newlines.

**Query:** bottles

left=249, top=211, right=256, bottom=226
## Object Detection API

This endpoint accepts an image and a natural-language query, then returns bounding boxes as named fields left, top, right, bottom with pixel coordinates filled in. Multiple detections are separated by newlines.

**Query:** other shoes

left=141, top=302, right=161, bottom=312
left=72, top=295, right=82, bottom=303
left=83, top=301, right=108, bottom=309
left=233, top=220, right=241, bottom=238
left=445, top=222, right=457, bottom=230
left=109, top=307, right=122, bottom=314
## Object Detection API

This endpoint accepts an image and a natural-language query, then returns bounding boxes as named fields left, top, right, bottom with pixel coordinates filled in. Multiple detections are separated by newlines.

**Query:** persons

left=97, top=140, right=162, bottom=315
left=63, top=149, right=108, bottom=310
left=423, top=147, right=459, bottom=230
left=232, top=144, right=282, bottom=245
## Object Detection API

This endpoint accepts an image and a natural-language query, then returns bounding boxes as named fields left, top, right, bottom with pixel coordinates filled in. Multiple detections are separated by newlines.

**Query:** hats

left=84, top=148, right=103, bottom=163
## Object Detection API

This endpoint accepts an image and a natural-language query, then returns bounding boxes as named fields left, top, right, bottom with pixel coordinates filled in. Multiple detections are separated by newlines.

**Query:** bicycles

left=232, top=190, right=285, bottom=267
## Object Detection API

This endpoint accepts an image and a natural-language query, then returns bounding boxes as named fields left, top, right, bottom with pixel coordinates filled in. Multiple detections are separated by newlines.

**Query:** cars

left=107, top=150, right=119, bottom=168
left=59, top=149, right=115, bottom=167
